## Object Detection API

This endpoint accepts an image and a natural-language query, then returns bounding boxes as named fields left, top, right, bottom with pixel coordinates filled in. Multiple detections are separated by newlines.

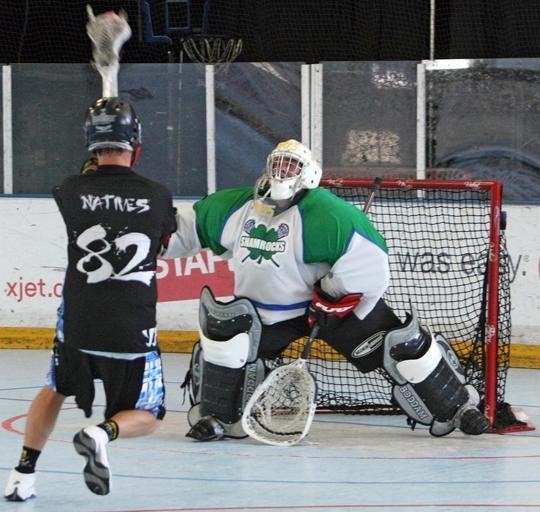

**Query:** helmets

left=267, top=138, right=324, bottom=201
left=84, top=97, right=143, bottom=152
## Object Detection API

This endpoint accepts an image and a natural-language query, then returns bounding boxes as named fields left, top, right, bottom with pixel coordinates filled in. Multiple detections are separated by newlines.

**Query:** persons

left=161, top=139, right=490, bottom=442
left=4, top=97, right=178, bottom=502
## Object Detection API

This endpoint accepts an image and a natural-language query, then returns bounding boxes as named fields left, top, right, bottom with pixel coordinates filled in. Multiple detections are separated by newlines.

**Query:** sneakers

left=183, top=419, right=218, bottom=443
left=458, top=406, right=491, bottom=434
left=3, top=466, right=37, bottom=501
left=71, top=424, right=113, bottom=496
